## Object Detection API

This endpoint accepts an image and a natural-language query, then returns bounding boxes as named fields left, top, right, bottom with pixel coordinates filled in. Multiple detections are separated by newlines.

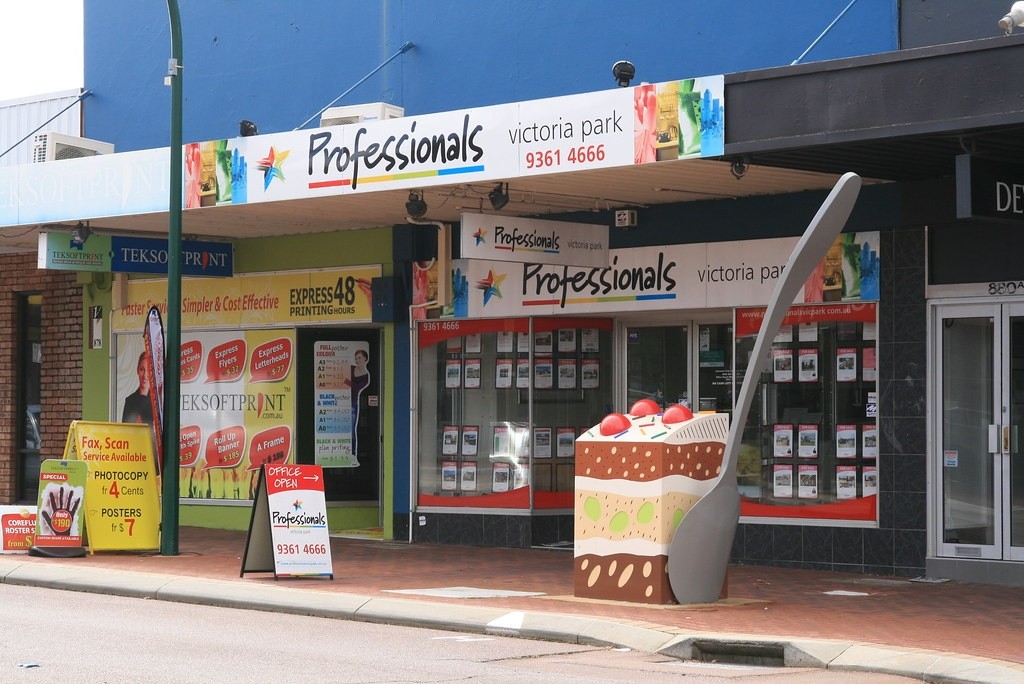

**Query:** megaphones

left=998, top=1, right=1024, bottom=28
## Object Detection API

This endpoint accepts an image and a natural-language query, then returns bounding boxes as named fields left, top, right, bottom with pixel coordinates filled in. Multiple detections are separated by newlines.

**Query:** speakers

left=372, top=275, right=406, bottom=323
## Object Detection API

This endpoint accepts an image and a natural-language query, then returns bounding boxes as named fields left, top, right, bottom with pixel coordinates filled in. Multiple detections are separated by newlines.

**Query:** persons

left=121, top=352, right=153, bottom=424
left=351, top=350, right=368, bottom=465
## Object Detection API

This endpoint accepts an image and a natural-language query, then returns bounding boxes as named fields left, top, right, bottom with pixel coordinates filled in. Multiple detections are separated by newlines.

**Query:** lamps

left=69, top=222, right=98, bottom=245
left=485, top=184, right=513, bottom=212
left=240, top=119, right=263, bottom=135
left=403, top=190, right=431, bottom=218
left=995, top=0, right=1024, bottom=36
left=611, top=59, right=639, bottom=85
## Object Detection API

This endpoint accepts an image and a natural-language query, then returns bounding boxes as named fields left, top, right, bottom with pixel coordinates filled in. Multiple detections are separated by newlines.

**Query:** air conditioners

left=319, top=101, right=405, bottom=126
left=32, top=132, right=119, bottom=162
